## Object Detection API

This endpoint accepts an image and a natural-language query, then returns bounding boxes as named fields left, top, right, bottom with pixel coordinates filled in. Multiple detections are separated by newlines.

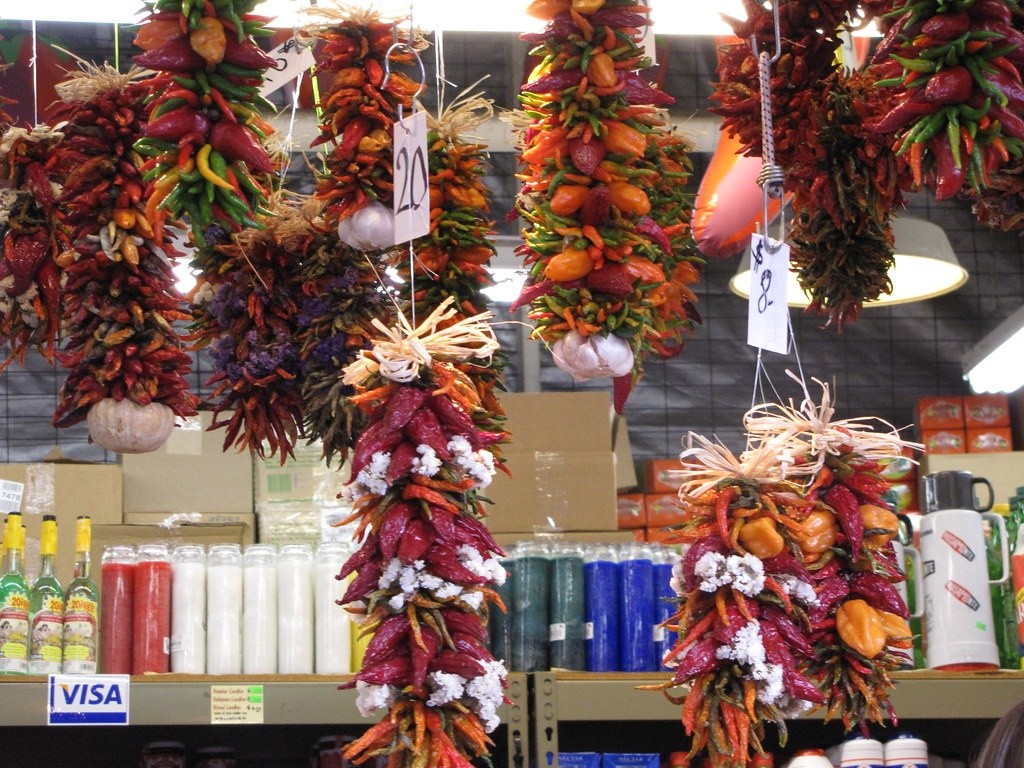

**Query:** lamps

left=961, top=304, right=1024, bottom=394
left=727, top=203, right=974, bottom=312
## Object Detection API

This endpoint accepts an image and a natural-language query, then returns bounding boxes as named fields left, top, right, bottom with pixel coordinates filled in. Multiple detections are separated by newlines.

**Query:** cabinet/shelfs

left=0, top=673, right=527, bottom=768
left=530, top=669, right=1024, bottom=768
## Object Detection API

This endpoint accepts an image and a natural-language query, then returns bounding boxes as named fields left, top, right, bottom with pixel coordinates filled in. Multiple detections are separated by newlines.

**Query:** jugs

left=917, top=470, right=1011, bottom=674
left=887, top=511, right=924, bottom=671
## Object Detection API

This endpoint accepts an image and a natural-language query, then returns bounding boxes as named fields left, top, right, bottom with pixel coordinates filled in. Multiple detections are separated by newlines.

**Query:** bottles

left=0, top=511, right=100, bottom=677
left=96, top=539, right=682, bottom=673
left=670, top=730, right=929, bottom=768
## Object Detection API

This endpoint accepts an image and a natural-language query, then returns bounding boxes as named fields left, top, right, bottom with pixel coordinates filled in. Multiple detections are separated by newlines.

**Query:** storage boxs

left=0, top=392, right=1024, bottom=673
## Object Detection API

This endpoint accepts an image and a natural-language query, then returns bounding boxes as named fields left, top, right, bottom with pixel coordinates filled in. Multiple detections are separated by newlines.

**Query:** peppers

left=0, top=0, right=1024, bottom=768
